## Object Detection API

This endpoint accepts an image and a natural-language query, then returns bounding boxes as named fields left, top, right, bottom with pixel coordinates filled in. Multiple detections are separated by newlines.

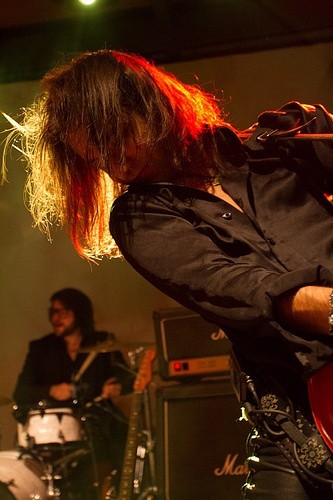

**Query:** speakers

left=153, top=306, right=233, bottom=383
left=155, top=381, right=250, bottom=500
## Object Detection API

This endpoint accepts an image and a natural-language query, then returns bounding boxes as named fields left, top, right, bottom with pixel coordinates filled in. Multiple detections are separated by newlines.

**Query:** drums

left=15, top=405, right=84, bottom=449
left=0, top=449, right=62, bottom=499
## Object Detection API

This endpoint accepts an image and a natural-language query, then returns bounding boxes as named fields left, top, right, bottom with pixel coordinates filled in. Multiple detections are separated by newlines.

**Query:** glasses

left=47, top=306, right=68, bottom=316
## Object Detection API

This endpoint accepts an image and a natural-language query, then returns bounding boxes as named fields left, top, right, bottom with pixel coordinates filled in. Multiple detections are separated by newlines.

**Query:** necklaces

left=169, top=171, right=220, bottom=186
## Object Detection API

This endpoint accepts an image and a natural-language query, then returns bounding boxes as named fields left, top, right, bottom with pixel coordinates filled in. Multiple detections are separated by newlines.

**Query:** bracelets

left=328, top=290, right=333, bottom=335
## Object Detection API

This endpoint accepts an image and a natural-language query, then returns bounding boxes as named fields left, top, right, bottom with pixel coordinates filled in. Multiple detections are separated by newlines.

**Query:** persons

left=23, top=50, right=333, bottom=500
left=11, top=288, right=136, bottom=500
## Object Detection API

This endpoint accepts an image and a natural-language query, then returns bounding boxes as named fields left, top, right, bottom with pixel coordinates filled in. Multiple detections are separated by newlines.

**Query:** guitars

left=305, top=370, right=333, bottom=457
left=116, top=346, right=159, bottom=500
